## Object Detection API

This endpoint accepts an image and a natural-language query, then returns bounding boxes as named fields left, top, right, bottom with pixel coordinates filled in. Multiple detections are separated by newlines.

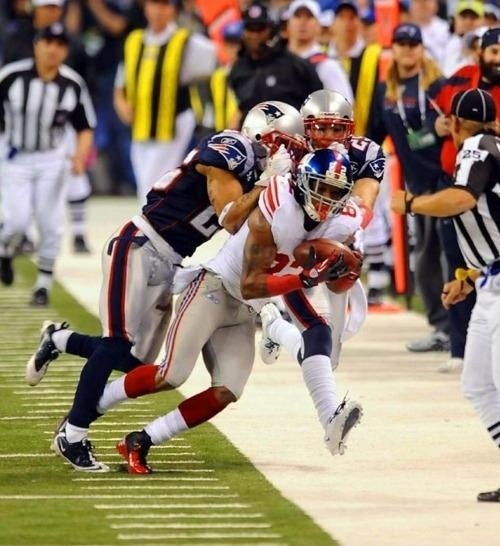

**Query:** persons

left=1, top=0, right=500, bottom=500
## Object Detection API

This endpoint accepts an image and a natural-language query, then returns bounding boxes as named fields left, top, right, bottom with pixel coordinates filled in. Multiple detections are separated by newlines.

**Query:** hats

left=449, top=87, right=496, bottom=124
left=332, top=0, right=358, bottom=16
left=391, top=23, right=424, bottom=45
left=481, top=27, right=500, bottom=49
left=35, top=21, right=72, bottom=45
left=286, top=0, right=322, bottom=21
left=455, top=1, right=486, bottom=17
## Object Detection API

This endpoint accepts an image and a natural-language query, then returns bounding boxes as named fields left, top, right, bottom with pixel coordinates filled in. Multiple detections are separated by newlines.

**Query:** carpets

left=1, top=254, right=339, bottom=545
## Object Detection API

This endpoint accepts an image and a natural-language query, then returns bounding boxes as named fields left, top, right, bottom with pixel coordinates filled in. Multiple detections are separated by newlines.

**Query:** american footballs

left=293, top=239, right=361, bottom=278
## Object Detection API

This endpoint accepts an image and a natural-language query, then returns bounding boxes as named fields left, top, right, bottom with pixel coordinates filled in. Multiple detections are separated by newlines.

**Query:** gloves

left=303, top=244, right=347, bottom=289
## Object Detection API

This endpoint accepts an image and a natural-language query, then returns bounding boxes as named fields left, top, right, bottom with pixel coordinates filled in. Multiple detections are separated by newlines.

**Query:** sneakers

left=29, top=284, right=50, bottom=305
left=116, top=436, right=153, bottom=474
left=51, top=413, right=111, bottom=474
left=0, top=256, right=14, bottom=285
left=325, top=399, right=363, bottom=461
left=259, top=304, right=281, bottom=367
left=25, top=318, right=64, bottom=388
left=407, top=329, right=452, bottom=353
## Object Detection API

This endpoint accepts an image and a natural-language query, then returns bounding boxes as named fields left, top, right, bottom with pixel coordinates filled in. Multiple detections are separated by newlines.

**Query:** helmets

left=241, top=87, right=354, bottom=220
